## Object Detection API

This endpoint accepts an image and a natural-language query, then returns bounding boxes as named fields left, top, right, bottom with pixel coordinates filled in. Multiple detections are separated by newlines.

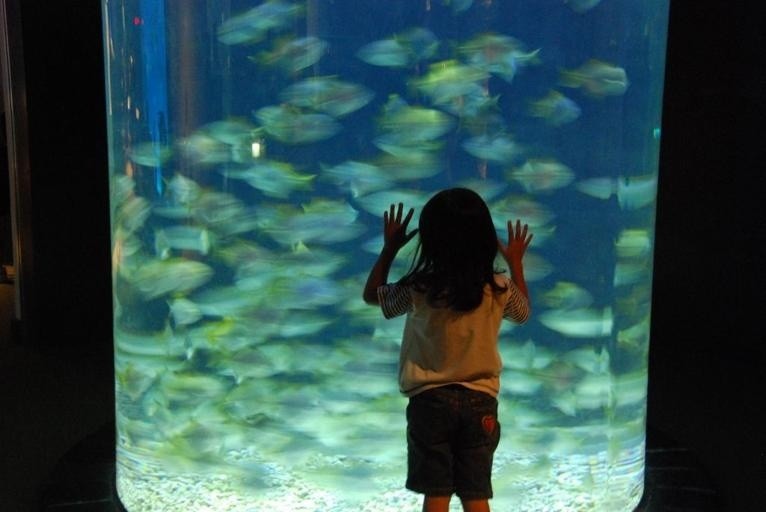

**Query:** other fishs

left=105, top=3, right=660, bottom=510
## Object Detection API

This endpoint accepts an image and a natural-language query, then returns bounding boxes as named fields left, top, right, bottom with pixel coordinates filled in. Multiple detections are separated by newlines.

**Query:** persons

left=362, top=187, right=534, bottom=511
